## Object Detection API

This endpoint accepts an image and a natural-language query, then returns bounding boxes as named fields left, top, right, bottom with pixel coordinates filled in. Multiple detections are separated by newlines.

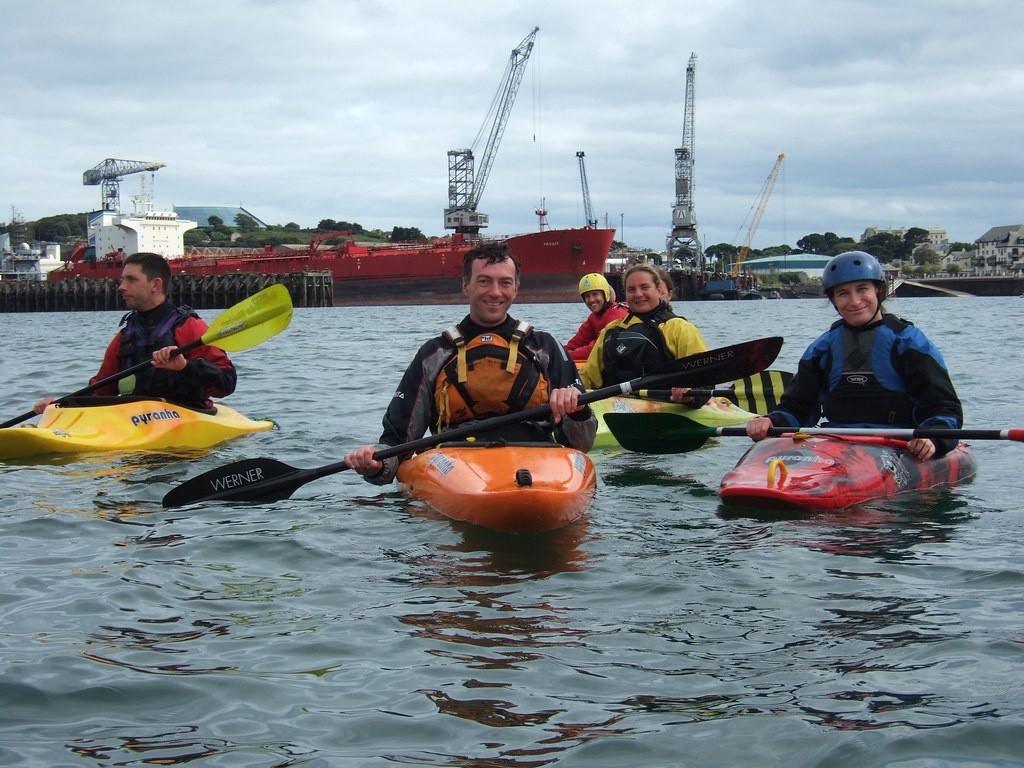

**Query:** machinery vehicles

left=705, top=153, right=785, bottom=299
left=664, top=51, right=708, bottom=275
left=443, top=26, right=542, bottom=242
left=83, top=155, right=166, bottom=213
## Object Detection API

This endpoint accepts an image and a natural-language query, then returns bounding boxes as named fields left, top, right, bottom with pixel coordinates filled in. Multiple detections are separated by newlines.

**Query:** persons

left=33, top=253, right=237, bottom=414
left=746, top=251, right=963, bottom=463
left=563, top=264, right=715, bottom=409
left=344, top=243, right=599, bottom=485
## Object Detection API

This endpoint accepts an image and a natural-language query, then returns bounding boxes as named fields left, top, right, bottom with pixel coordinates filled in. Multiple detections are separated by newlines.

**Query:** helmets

left=822, top=251, right=885, bottom=293
left=579, top=272, right=610, bottom=303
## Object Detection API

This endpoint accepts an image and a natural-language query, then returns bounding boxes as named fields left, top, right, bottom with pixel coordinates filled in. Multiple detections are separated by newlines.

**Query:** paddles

left=588, top=370, right=793, bottom=417
left=602, top=412, right=1023, bottom=458
left=162, top=335, right=784, bottom=507
left=0, top=283, right=294, bottom=428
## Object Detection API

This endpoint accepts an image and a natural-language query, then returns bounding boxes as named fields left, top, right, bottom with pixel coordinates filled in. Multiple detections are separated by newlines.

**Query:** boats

left=716, top=425, right=978, bottom=519
left=396, top=436, right=597, bottom=533
left=553, top=387, right=765, bottom=450
left=44, top=153, right=620, bottom=297
left=0, top=394, right=274, bottom=465
